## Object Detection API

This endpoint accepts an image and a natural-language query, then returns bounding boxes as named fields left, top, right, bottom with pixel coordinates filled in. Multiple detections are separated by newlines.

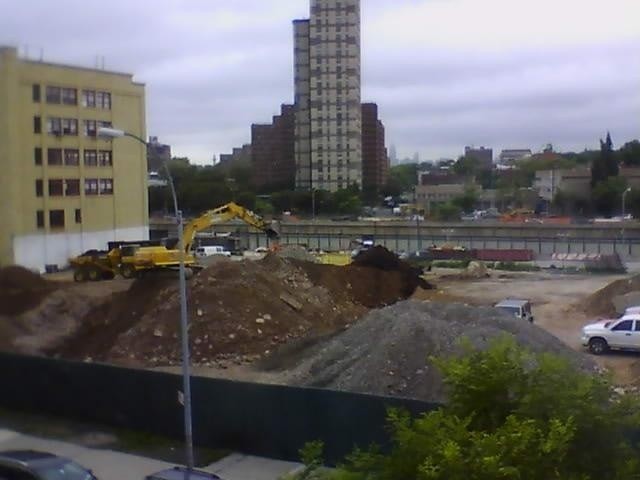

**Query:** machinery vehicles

left=116, top=202, right=283, bottom=279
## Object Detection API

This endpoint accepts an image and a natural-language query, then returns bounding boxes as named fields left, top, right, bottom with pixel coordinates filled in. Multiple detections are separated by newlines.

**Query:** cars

left=2, top=448, right=221, bottom=480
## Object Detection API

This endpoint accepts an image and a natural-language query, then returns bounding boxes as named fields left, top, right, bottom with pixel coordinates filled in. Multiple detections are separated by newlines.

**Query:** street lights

left=621, top=187, right=632, bottom=242
left=98, top=125, right=197, bottom=471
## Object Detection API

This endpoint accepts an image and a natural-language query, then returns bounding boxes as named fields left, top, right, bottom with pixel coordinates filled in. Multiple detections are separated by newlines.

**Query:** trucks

left=68, top=244, right=139, bottom=283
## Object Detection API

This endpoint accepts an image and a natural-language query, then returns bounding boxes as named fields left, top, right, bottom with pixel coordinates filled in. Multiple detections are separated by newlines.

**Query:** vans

left=494, top=299, right=533, bottom=321
left=194, top=245, right=231, bottom=259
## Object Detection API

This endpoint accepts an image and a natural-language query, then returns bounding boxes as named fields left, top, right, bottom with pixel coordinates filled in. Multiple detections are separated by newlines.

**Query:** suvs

left=582, top=305, right=639, bottom=357
